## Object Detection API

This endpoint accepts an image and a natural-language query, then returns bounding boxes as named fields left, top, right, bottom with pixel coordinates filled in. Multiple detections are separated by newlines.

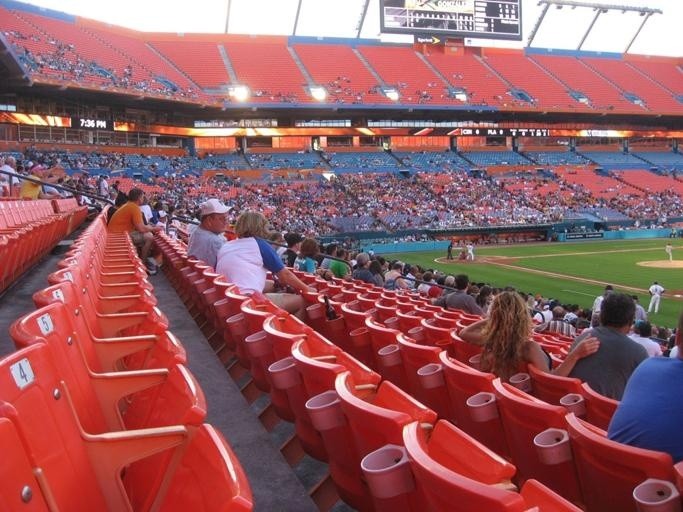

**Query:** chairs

left=446, top=272, right=683, bottom=512
left=0, top=196, right=88, bottom=293
left=434, top=231, right=549, bottom=246
left=154, top=225, right=446, bottom=512
left=361, top=237, right=451, bottom=253
left=1, top=204, right=153, bottom=512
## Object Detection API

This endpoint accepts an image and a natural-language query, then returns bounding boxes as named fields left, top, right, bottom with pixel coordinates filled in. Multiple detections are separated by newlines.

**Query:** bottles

left=324, top=295, right=338, bottom=321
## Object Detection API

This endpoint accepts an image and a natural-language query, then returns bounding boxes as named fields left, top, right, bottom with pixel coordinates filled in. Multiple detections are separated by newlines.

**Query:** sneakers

left=144, top=264, right=159, bottom=275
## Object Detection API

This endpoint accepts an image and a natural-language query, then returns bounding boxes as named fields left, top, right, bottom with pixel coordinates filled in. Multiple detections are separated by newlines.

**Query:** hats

left=201, top=198, right=232, bottom=217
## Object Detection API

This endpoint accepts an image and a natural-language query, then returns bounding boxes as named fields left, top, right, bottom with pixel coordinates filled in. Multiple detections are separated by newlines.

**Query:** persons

left=294, top=239, right=320, bottom=275
left=468, top=270, right=579, bottom=322
left=566, top=294, right=648, bottom=401
left=577, top=309, right=590, bottom=328
left=0, top=149, right=189, bottom=230
left=607, top=314, right=683, bottom=464
left=188, top=199, right=232, bottom=270
left=214, top=214, right=317, bottom=325
left=280, top=233, right=306, bottom=269
left=459, top=251, right=466, bottom=260
left=665, top=244, right=673, bottom=261
left=321, top=242, right=456, bottom=297
left=459, top=291, right=602, bottom=380
left=535, top=306, right=576, bottom=340
left=652, top=321, right=678, bottom=348
left=648, top=280, right=664, bottom=313
left=633, top=323, right=663, bottom=356
left=590, top=285, right=614, bottom=327
left=467, top=244, right=474, bottom=261
left=108, top=189, right=162, bottom=275
left=631, top=296, right=648, bottom=329
left=432, top=274, right=487, bottom=319
